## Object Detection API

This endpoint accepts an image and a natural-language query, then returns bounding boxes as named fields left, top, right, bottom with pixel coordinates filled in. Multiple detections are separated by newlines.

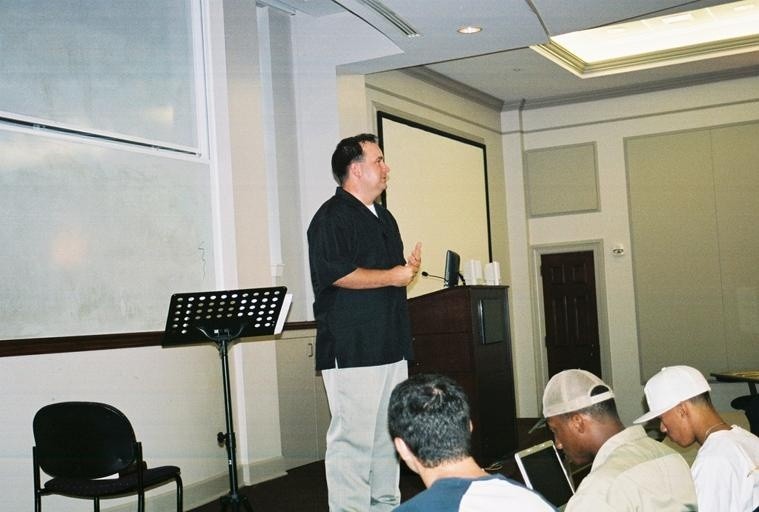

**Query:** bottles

left=528, top=369, right=616, bottom=435
left=633, top=364, right=711, bottom=425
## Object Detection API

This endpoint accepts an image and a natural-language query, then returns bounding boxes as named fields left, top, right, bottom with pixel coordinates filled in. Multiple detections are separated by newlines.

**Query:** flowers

left=421, top=271, right=445, bottom=280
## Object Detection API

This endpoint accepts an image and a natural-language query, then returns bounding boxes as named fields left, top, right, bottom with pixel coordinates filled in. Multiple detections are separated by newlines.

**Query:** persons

left=640, top=365, right=757, bottom=512
left=532, top=369, right=701, bottom=512
left=389, top=372, right=556, bottom=512
left=307, top=133, right=422, bottom=512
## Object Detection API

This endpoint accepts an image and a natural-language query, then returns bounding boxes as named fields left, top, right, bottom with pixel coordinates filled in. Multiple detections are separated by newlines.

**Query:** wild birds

left=513, top=439, right=575, bottom=512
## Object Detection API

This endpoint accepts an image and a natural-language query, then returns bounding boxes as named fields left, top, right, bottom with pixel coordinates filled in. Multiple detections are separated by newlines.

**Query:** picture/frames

left=709, top=370, right=758, bottom=436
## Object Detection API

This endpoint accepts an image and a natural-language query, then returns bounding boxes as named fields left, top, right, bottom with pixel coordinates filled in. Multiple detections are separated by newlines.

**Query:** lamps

left=33, top=402, right=183, bottom=512
left=729, top=394, right=759, bottom=438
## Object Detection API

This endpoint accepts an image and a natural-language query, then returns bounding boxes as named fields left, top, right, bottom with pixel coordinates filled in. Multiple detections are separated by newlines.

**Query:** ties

left=443, top=249, right=461, bottom=289
